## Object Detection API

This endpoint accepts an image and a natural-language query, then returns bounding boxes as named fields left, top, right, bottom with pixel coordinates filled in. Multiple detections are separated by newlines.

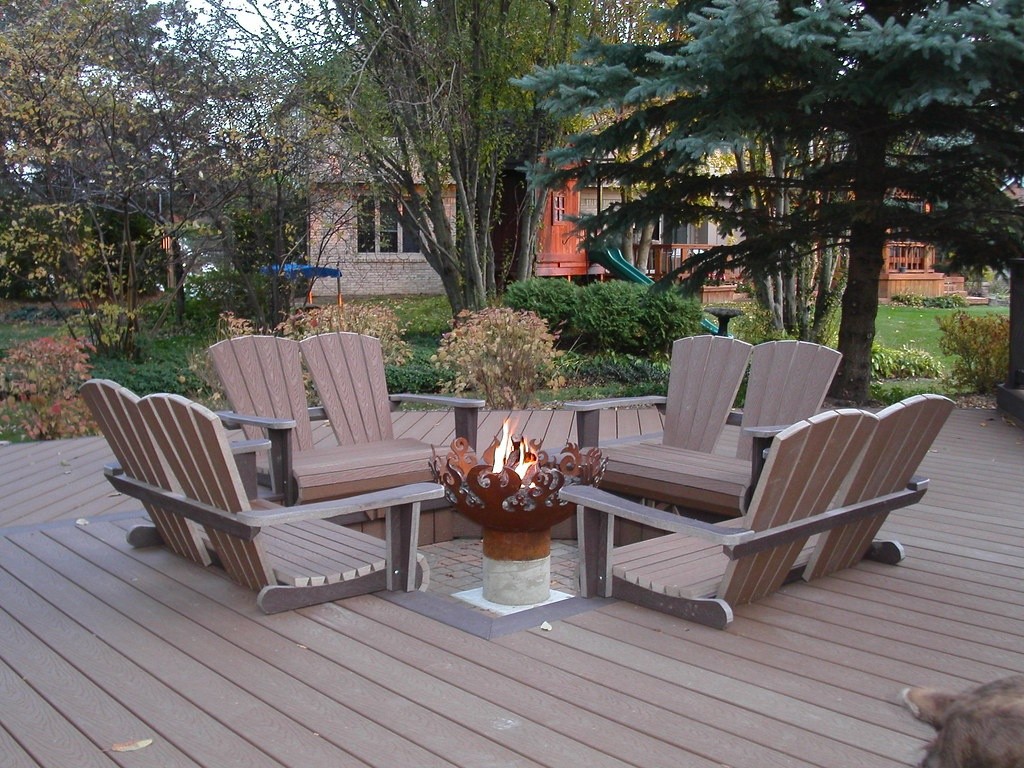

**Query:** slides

left=591, top=240, right=735, bottom=339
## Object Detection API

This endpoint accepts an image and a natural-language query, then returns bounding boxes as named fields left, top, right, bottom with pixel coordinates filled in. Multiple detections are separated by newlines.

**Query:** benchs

left=77, top=377, right=446, bottom=617
left=206, top=331, right=488, bottom=510
left=552, top=393, right=959, bottom=630
left=569, top=334, right=843, bottom=516
